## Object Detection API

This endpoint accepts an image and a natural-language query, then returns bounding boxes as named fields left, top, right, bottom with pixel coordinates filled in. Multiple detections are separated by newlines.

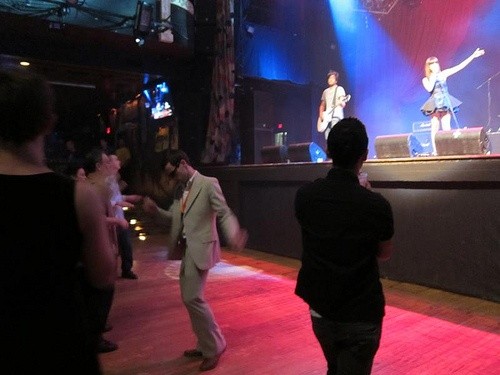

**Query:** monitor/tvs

left=142, top=81, right=176, bottom=124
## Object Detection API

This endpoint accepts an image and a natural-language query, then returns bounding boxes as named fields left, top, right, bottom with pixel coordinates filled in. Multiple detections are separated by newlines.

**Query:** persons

left=293, top=116, right=394, bottom=375
left=136, top=149, right=247, bottom=372
left=0, top=63, right=143, bottom=375
left=319, top=70, right=346, bottom=152
left=420, top=47, right=486, bottom=155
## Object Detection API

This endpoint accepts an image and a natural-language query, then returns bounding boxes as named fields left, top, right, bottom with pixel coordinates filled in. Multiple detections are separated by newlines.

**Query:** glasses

left=169, top=167, right=178, bottom=177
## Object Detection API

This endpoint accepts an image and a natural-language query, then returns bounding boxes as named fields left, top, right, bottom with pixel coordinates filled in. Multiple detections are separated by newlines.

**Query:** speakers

left=434, top=127, right=492, bottom=156
left=374, top=134, right=425, bottom=159
left=260, top=142, right=327, bottom=163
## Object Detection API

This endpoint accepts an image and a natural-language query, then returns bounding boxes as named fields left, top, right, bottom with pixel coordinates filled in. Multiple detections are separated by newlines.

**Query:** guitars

left=317, top=94, right=352, bottom=133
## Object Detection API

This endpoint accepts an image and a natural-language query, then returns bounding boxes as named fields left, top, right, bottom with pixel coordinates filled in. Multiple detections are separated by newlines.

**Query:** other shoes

left=122, top=270, right=137, bottom=279
left=186, top=349, right=202, bottom=357
left=100, top=339, right=118, bottom=352
left=103, top=323, right=112, bottom=332
left=200, top=345, right=227, bottom=371
